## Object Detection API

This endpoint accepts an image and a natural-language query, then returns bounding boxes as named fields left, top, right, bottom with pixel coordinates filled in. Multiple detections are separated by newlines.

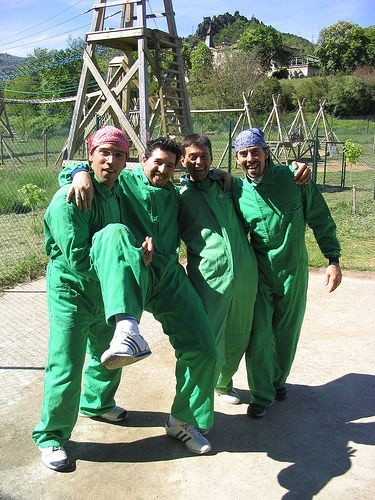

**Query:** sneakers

left=100, top=334, right=152, bottom=370
left=79, top=406, right=128, bottom=422
left=200, top=428, right=209, bottom=436
left=275, top=388, right=288, bottom=401
left=166, top=416, right=211, bottom=454
left=247, top=402, right=266, bottom=419
left=216, top=387, right=240, bottom=405
left=38, top=446, right=69, bottom=470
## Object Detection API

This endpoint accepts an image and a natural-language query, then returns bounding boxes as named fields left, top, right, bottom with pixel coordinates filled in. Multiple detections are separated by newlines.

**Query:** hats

left=235, top=128, right=270, bottom=154
left=87, top=126, right=130, bottom=153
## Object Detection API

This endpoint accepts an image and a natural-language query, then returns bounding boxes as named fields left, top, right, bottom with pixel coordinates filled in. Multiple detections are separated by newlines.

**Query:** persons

left=176, top=133, right=313, bottom=436
left=33, top=126, right=154, bottom=470
left=59, top=137, right=236, bottom=454
left=232, top=129, right=342, bottom=419
